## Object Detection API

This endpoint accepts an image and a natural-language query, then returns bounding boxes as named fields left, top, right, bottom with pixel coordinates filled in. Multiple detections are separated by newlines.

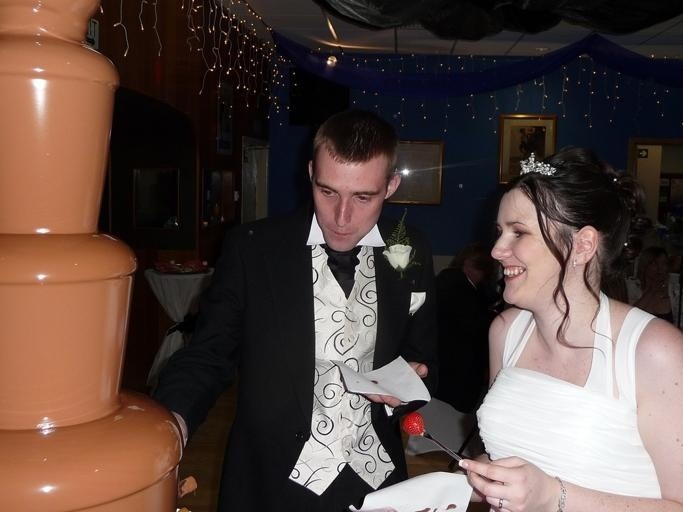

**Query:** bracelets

left=553, top=476, right=566, bottom=512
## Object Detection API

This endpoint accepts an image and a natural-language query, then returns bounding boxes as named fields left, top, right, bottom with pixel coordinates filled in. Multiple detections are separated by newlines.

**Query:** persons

left=484, top=219, right=683, bottom=331
left=408, top=234, right=498, bottom=417
left=147, top=105, right=440, bottom=511
left=450, top=140, right=683, bottom=512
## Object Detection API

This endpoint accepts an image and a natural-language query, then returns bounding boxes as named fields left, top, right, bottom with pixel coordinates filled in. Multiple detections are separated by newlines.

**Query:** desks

left=138, top=261, right=214, bottom=400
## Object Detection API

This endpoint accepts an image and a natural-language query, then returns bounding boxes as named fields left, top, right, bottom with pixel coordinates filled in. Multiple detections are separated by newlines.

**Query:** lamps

left=310, top=48, right=342, bottom=69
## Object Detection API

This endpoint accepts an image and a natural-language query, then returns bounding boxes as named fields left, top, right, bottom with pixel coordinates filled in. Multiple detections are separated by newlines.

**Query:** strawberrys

left=402, top=412, right=424, bottom=436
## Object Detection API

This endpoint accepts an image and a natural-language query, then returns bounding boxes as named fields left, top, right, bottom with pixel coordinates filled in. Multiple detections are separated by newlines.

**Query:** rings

left=498, top=497, right=504, bottom=509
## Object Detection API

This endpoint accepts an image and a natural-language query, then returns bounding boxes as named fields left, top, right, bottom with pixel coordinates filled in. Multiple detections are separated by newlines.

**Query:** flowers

left=382, top=209, right=424, bottom=283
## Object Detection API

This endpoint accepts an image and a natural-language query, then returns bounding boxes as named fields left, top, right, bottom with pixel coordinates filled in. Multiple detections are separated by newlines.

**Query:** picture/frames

left=382, top=136, right=446, bottom=208
left=495, top=111, right=558, bottom=188
left=237, top=134, right=273, bottom=228
